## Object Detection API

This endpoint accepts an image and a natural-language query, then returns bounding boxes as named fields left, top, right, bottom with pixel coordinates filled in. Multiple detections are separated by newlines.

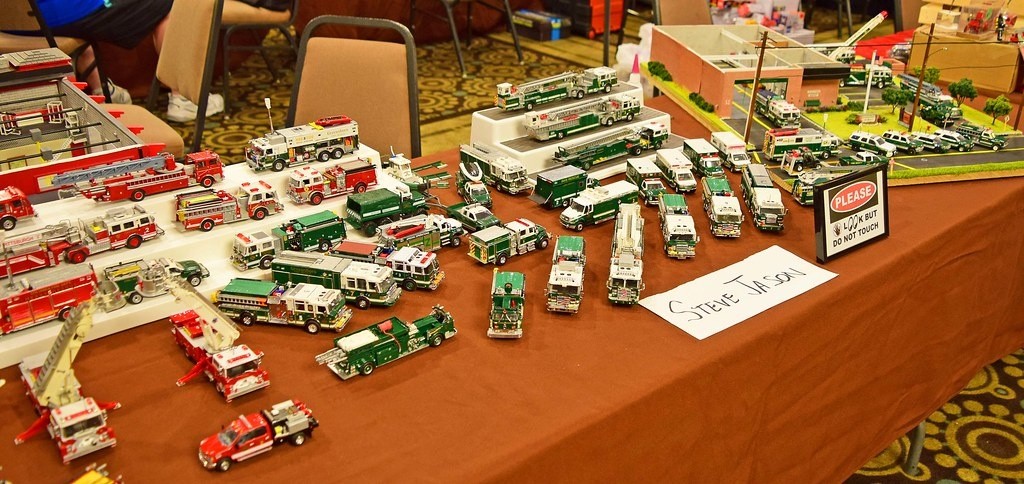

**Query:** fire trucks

left=710, top=131, right=752, bottom=172
left=656, top=148, right=697, bottom=193
left=790, top=169, right=858, bottom=206
left=216, top=277, right=353, bottom=334
left=228, top=211, right=347, bottom=273
left=466, top=218, right=552, bottom=266
left=377, top=214, right=468, bottom=255
left=804, top=43, right=858, bottom=63
left=459, top=142, right=535, bottom=195
left=896, top=73, right=965, bottom=120
left=545, top=232, right=585, bottom=314
left=701, top=175, right=745, bottom=239
left=242, top=111, right=360, bottom=172
left=740, top=163, right=788, bottom=232
left=101, top=256, right=211, bottom=313
left=963, top=10, right=994, bottom=35
left=494, top=65, right=619, bottom=112
left=0, top=267, right=102, bottom=339
left=656, top=192, right=701, bottom=259
left=272, top=250, right=404, bottom=312
left=762, top=126, right=843, bottom=161
left=51, top=150, right=225, bottom=203
left=487, top=267, right=526, bottom=339
left=325, top=240, right=446, bottom=292
left=828, top=11, right=895, bottom=89
left=197, top=397, right=320, bottom=473
left=67, top=464, right=128, bottom=484
left=0, top=205, right=166, bottom=281
left=12, top=281, right=128, bottom=467
left=683, top=138, right=725, bottom=177
left=314, top=303, right=458, bottom=380
left=955, top=122, right=1010, bottom=152
left=754, top=90, right=802, bottom=128
left=605, top=202, right=644, bottom=305
left=524, top=93, right=643, bottom=141
left=171, top=178, right=284, bottom=233
left=455, top=170, right=494, bottom=207
left=137, top=265, right=273, bottom=402
left=0, top=184, right=41, bottom=233
left=558, top=179, right=640, bottom=232
left=552, top=120, right=671, bottom=171
left=626, top=155, right=667, bottom=206
left=288, top=156, right=378, bottom=206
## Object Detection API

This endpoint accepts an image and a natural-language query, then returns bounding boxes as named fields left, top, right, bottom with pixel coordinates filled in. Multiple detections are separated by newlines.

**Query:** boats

left=387, top=223, right=425, bottom=238
left=316, top=115, right=351, bottom=126
left=460, top=161, right=482, bottom=183
left=383, top=146, right=431, bottom=194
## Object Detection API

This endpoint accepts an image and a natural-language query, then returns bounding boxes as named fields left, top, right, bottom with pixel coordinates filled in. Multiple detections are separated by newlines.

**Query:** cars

left=839, top=150, right=889, bottom=168
left=446, top=200, right=502, bottom=232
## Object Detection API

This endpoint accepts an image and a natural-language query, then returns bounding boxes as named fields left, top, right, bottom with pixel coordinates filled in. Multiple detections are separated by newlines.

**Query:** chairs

left=285, top=15, right=430, bottom=156
left=651, top=0, right=714, bottom=26
left=220, top=0, right=299, bottom=83
left=893, top=0, right=923, bottom=33
left=100, top=0, right=225, bottom=164
left=0, top=0, right=112, bottom=103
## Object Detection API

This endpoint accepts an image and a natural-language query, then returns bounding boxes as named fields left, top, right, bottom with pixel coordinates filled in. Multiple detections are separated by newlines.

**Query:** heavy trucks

left=528, top=166, right=601, bottom=209
left=344, top=172, right=431, bottom=237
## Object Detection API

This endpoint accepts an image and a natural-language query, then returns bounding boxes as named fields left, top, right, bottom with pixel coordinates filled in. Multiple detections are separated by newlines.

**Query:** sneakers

left=167, top=91, right=224, bottom=122
left=92, top=78, right=133, bottom=104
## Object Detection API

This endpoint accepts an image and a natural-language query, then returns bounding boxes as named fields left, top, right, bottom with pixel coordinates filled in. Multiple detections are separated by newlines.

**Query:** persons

left=0, top=0, right=224, bottom=122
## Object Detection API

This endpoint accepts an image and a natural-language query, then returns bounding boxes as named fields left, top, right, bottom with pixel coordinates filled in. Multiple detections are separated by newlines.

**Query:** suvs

left=911, top=133, right=951, bottom=154
left=919, top=102, right=955, bottom=127
left=882, top=130, right=924, bottom=157
left=934, top=129, right=974, bottom=150
left=849, top=131, right=897, bottom=157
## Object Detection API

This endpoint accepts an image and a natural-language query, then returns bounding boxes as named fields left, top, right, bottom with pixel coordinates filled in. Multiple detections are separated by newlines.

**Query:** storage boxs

left=907, top=24, right=1024, bottom=91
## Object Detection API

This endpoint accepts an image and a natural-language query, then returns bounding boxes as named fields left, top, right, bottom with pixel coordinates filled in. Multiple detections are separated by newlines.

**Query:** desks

left=0, top=29, right=1024, bottom=484
